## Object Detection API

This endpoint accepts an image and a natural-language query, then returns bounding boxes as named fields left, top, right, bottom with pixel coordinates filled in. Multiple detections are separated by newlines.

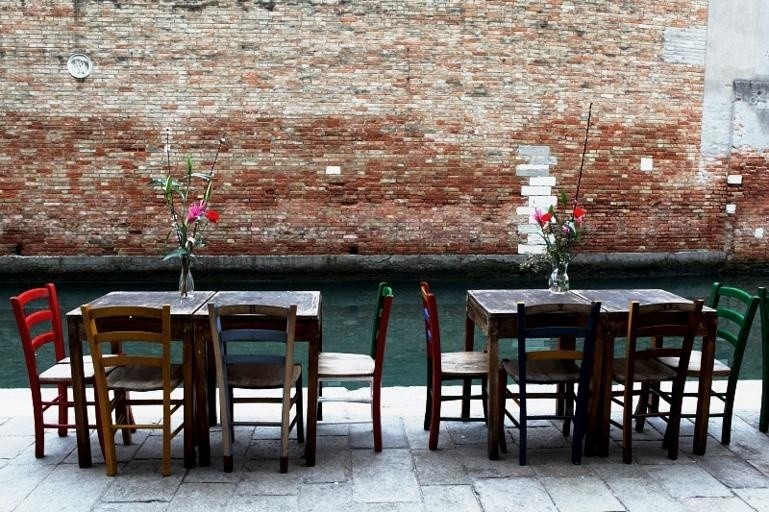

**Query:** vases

left=548, top=260, right=570, bottom=295
left=178, top=243, right=195, bottom=300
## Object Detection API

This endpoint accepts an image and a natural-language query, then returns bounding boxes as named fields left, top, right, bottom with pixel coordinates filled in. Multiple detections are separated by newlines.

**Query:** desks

left=462, top=288, right=719, bottom=461
left=65, top=290, right=323, bottom=468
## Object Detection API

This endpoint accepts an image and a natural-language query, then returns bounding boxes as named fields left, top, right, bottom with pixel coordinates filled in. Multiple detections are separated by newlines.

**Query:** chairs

left=635, top=281, right=761, bottom=446
left=502, top=300, right=602, bottom=466
left=420, top=281, right=508, bottom=454
left=612, top=298, right=705, bottom=464
left=756, top=285, right=769, bottom=433
left=8, top=282, right=126, bottom=459
left=207, top=302, right=305, bottom=473
left=80, top=303, right=197, bottom=477
left=318, top=281, right=394, bottom=453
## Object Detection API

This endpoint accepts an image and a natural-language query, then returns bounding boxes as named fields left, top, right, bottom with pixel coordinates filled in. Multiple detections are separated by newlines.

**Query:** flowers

left=151, top=122, right=226, bottom=298
left=517, top=101, right=616, bottom=290
